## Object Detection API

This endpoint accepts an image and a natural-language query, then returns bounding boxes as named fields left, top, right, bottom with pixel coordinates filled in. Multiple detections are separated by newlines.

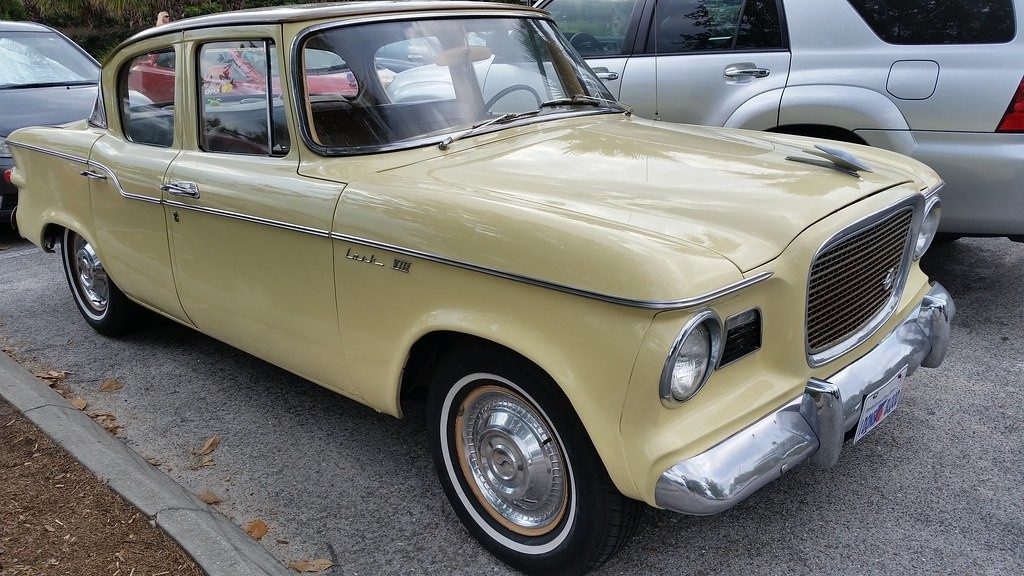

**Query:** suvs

left=381, top=2, right=1024, bottom=244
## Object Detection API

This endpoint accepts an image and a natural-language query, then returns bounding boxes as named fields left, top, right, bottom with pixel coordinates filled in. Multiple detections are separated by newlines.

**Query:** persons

left=157, top=11, right=169, bottom=26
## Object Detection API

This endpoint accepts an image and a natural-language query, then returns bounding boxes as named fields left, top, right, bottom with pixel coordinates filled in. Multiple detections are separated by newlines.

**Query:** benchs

left=129, top=101, right=357, bottom=147
left=203, top=100, right=487, bottom=155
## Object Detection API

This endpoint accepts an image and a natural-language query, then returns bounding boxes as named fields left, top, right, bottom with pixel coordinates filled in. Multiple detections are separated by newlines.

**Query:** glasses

left=165, top=15, right=169, bottom=18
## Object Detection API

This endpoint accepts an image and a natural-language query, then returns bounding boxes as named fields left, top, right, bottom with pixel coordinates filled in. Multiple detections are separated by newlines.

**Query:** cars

left=4, top=1, right=961, bottom=576
left=128, top=43, right=398, bottom=105
left=0, top=18, right=160, bottom=198
left=373, top=28, right=489, bottom=73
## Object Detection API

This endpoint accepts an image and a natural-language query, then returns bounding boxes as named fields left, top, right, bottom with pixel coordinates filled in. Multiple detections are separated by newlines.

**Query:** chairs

left=659, top=3, right=711, bottom=54
